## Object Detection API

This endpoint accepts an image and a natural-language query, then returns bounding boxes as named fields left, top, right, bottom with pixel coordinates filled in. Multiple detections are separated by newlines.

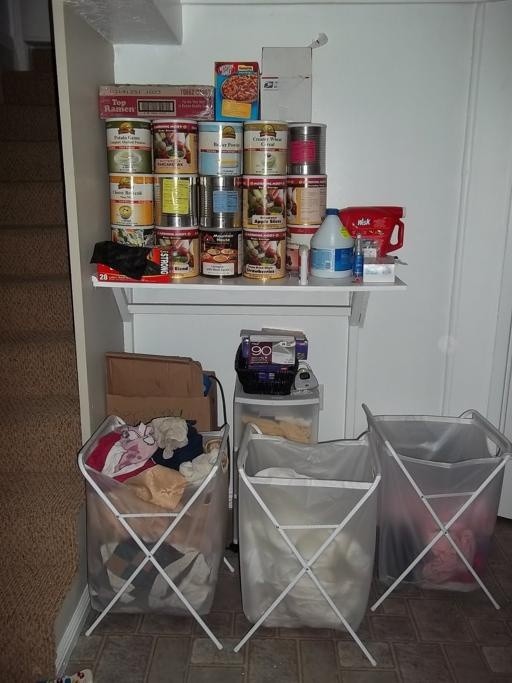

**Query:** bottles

left=352, top=232, right=366, bottom=280
left=296, top=244, right=310, bottom=286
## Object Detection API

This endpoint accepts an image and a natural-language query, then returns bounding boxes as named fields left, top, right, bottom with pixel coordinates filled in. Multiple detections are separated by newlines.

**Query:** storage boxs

left=97, top=81, right=215, bottom=121
left=105, top=348, right=219, bottom=431
left=233, top=379, right=320, bottom=545
left=259, top=46, right=312, bottom=125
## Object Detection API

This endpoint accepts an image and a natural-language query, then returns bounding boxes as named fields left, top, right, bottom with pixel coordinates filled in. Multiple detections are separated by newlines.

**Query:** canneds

left=104, top=117, right=328, bottom=281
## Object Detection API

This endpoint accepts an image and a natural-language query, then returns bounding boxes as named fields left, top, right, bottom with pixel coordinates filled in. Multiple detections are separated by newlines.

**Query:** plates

left=201, top=246, right=234, bottom=263
